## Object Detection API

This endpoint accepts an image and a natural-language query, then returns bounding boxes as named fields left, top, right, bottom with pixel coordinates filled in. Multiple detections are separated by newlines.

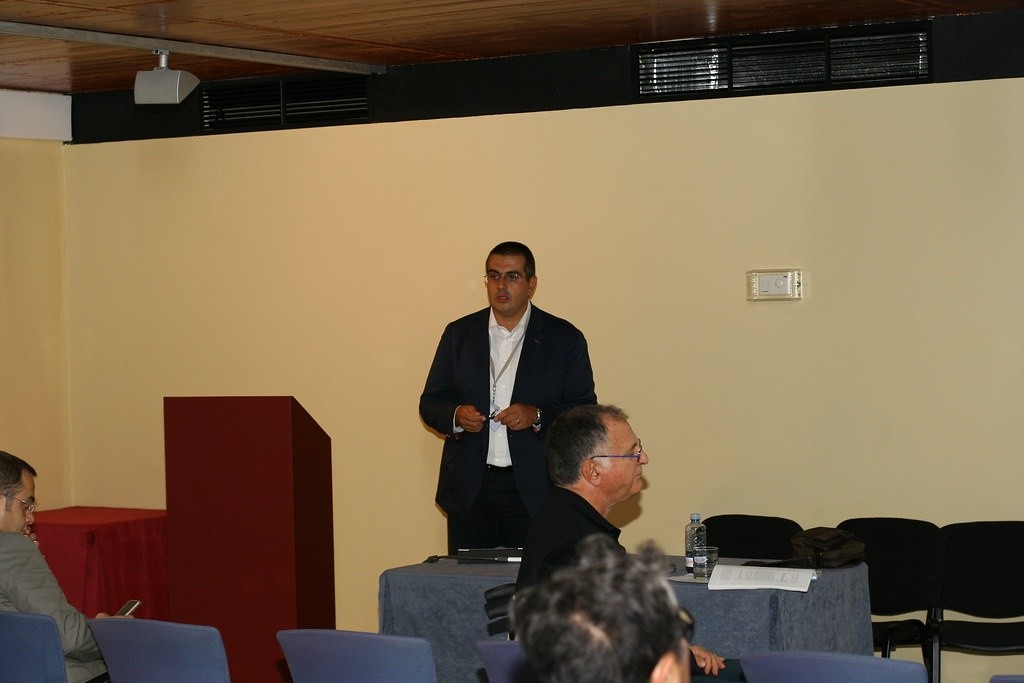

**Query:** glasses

left=590, top=439, right=642, bottom=463
left=484, top=273, right=530, bottom=282
left=1, top=492, right=36, bottom=513
left=676, top=607, right=695, bottom=643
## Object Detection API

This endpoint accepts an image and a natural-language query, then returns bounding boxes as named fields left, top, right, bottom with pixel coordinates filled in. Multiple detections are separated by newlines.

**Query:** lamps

left=132, top=51, right=199, bottom=106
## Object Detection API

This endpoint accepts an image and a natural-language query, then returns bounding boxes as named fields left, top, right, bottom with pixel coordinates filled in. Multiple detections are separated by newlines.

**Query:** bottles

left=686, top=513, right=707, bottom=577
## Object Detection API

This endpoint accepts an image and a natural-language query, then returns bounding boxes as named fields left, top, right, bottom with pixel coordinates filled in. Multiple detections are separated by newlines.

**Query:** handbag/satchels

left=791, top=527, right=866, bottom=568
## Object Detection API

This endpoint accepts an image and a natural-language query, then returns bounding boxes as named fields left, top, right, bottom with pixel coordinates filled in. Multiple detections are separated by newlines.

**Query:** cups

left=692, top=547, right=718, bottom=580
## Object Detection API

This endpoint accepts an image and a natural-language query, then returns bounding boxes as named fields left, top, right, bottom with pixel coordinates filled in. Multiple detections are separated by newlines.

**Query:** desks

left=27, top=506, right=168, bottom=620
left=375, top=554, right=875, bottom=683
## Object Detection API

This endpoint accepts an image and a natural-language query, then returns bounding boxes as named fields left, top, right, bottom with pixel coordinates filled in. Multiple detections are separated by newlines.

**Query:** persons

left=0, top=450, right=135, bottom=683
left=508, top=533, right=691, bottom=683
left=418, top=241, right=599, bottom=555
left=510, top=403, right=747, bottom=683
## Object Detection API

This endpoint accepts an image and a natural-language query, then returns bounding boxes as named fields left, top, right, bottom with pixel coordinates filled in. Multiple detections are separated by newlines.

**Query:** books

left=707, top=563, right=822, bottom=593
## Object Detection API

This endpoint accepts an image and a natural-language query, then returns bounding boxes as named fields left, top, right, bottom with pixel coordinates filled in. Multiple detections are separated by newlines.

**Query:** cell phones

left=115, top=600, right=142, bottom=616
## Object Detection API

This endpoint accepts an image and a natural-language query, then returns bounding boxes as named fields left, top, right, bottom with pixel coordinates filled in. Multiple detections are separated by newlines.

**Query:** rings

left=516, top=419, right=521, bottom=424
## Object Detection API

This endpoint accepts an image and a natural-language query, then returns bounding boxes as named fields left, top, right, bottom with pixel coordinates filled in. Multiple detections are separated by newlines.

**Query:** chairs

left=696, top=514, right=804, bottom=559
left=276, top=628, right=439, bottom=683
left=0, top=610, right=68, bottom=683
left=85, top=618, right=229, bottom=683
left=835, top=518, right=939, bottom=678
left=738, top=651, right=927, bottom=683
left=930, top=520, right=1024, bottom=683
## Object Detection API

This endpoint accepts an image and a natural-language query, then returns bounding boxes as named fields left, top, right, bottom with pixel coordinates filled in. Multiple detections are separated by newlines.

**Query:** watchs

left=536, top=406, right=543, bottom=427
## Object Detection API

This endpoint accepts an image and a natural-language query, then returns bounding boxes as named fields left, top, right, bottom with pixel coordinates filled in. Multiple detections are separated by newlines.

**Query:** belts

left=485, top=464, right=512, bottom=473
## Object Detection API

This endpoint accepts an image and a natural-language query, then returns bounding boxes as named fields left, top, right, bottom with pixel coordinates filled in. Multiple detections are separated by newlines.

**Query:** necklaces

left=489, top=299, right=532, bottom=403
